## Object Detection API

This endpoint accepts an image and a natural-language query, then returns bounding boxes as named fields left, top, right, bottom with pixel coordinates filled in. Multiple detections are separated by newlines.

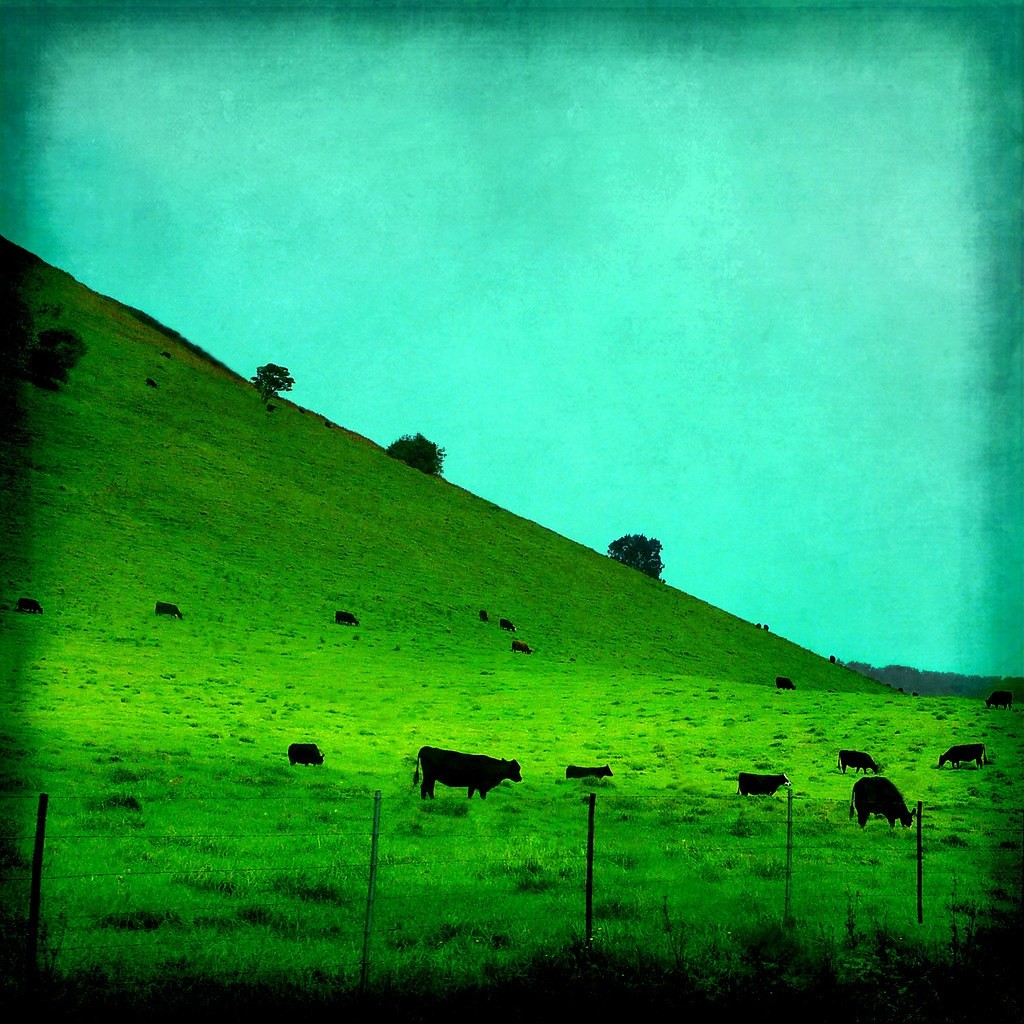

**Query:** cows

left=140, top=347, right=334, bottom=436
left=564, top=765, right=615, bottom=781
left=16, top=596, right=44, bottom=616
left=938, top=743, right=987, bottom=769
left=829, top=655, right=836, bottom=664
left=837, top=749, right=879, bottom=774
left=478, top=610, right=533, bottom=656
left=154, top=601, right=184, bottom=623
left=775, top=676, right=796, bottom=690
left=736, top=773, right=791, bottom=796
left=754, top=622, right=770, bottom=632
left=885, top=682, right=920, bottom=697
left=984, top=691, right=1014, bottom=710
left=285, top=742, right=327, bottom=768
left=849, top=776, right=916, bottom=828
left=334, top=608, right=362, bottom=628
left=412, top=746, right=522, bottom=799
